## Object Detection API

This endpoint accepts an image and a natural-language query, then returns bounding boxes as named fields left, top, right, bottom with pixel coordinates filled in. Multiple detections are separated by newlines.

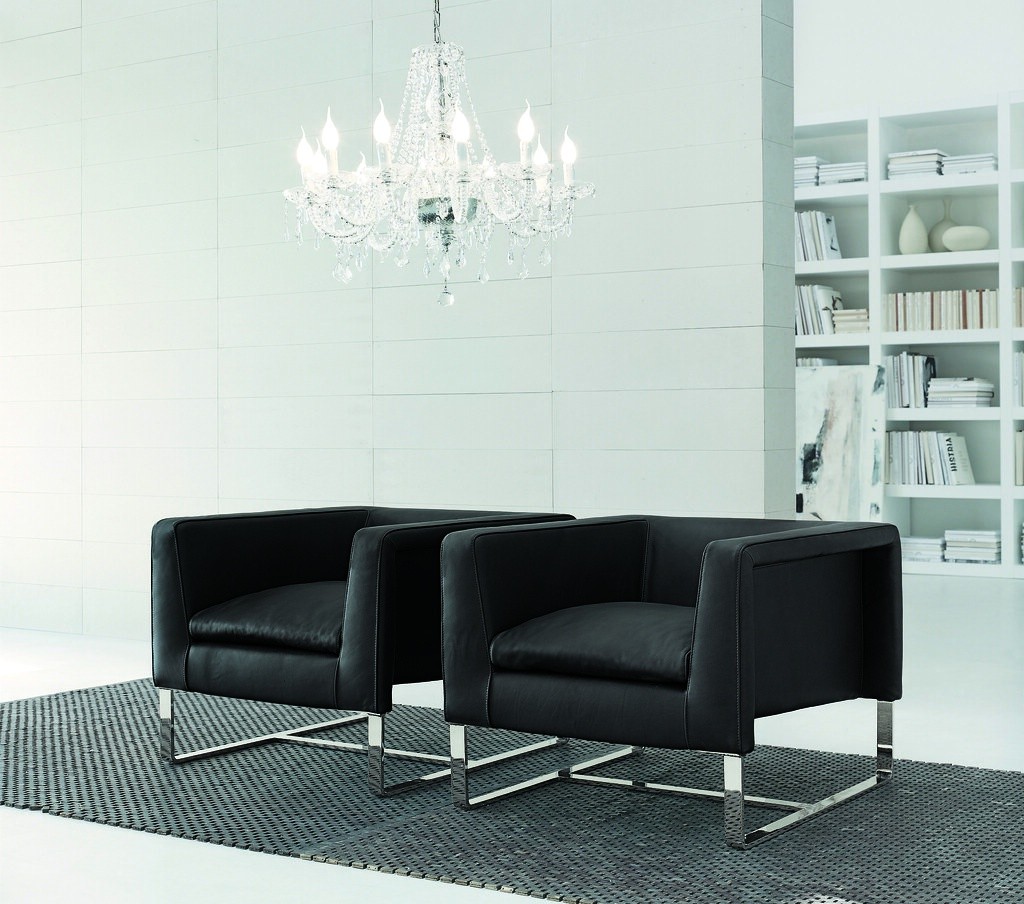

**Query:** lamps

left=282, top=0, right=594, bottom=307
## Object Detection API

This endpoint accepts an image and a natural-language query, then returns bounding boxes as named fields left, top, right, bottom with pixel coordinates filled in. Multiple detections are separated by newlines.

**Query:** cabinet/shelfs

left=790, top=90, right=1024, bottom=580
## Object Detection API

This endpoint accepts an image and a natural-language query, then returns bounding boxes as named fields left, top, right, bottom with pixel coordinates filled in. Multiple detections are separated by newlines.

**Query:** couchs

left=151, top=505, right=578, bottom=798
left=440, top=514, right=903, bottom=852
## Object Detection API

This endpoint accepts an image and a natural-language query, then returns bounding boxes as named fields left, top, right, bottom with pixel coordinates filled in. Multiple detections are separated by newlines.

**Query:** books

left=885, top=431, right=975, bottom=485
left=888, top=289, right=999, bottom=332
left=793, top=210, right=842, bottom=262
left=1015, top=287, right=1024, bottom=562
left=887, top=149, right=998, bottom=180
left=794, top=149, right=866, bottom=186
left=901, top=529, right=1001, bottom=564
left=798, top=357, right=838, bottom=367
left=887, top=351, right=994, bottom=409
left=794, top=283, right=869, bottom=334
